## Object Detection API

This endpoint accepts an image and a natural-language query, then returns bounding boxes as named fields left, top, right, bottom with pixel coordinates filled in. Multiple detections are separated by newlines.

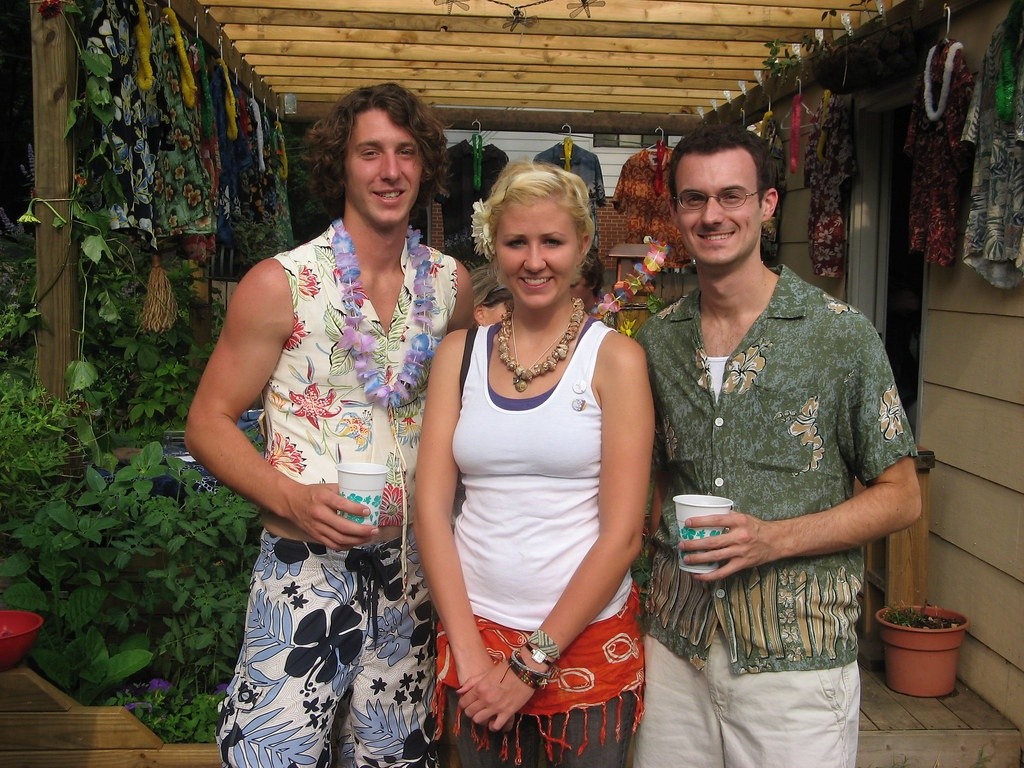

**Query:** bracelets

left=500, top=630, right=560, bottom=689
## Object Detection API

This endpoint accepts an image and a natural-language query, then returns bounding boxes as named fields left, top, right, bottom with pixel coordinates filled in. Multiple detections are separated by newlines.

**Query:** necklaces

left=330, top=218, right=444, bottom=409
left=497, top=295, right=585, bottom=392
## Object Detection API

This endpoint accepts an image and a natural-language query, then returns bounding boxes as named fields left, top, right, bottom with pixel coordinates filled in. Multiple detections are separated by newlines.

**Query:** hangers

left=466, top=5, right=974, bottom=165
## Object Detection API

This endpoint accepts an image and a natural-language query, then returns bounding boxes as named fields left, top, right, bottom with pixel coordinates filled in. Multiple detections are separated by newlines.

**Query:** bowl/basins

left=0, top=610, right=43, bottom=668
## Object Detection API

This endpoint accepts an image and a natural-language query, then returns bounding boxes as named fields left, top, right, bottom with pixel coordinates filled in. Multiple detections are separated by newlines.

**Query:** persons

left=628, top=125, right=923, bottom=768
left=413, top=160, right=654, bottom=768
left=184, top=80, right=476, bottom=768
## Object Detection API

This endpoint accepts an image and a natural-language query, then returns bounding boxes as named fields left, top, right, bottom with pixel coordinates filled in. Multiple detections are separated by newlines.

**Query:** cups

left=335, top=463, right=390, bottom=527
left=673, top=494, right=734, bottom=573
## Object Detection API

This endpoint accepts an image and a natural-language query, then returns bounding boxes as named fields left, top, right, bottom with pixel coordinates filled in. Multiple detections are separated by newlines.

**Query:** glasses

left=670, top=188, right=763, bottom=211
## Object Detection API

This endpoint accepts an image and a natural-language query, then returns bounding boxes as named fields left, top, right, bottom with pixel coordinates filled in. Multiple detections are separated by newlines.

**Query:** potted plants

left=875, top=601, right=971, bottom=697
